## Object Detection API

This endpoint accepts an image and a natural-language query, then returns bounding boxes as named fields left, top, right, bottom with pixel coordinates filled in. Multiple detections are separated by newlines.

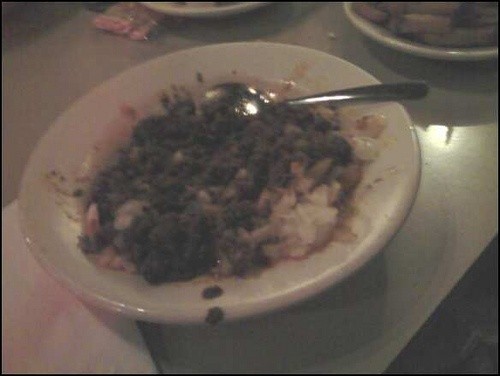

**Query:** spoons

left=200, top=81, right=429, bottom=130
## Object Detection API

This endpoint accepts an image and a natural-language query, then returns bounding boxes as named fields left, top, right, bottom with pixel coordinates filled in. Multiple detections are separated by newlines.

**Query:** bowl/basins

left=344, top=2, right=498, bottom=61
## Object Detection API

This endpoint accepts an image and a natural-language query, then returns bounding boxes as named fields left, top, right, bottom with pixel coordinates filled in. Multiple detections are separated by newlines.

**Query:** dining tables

left=0, top=0, right=500, bottom=375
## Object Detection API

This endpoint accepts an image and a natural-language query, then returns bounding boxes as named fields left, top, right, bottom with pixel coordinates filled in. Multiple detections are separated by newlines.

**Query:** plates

left=16, top=40, right=421, bottom=327
left=139, top=2, right=272, bottom=19
